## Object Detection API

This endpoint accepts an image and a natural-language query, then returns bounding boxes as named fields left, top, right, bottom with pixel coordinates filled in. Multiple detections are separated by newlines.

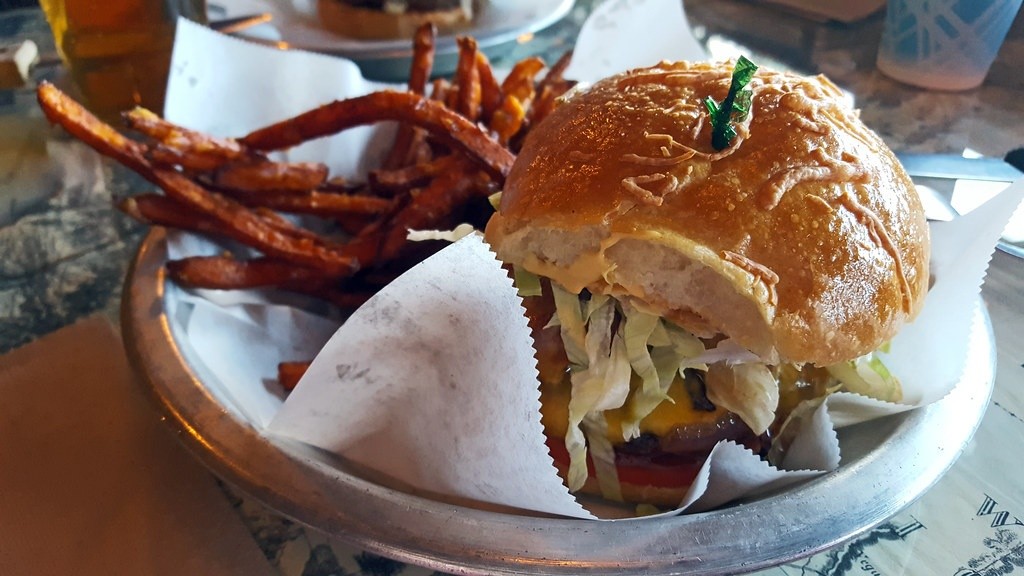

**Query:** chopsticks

left=207, top=12, right=288, bottom=50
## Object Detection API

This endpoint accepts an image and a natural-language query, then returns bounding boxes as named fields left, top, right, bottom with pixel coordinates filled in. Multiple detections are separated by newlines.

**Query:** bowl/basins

left=207, top=0, right=578, bottom=81
left=117, top=194, right=999, bottom=576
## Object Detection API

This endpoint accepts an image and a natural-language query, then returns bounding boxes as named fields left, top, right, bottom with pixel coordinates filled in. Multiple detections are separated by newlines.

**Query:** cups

left=875, top=1, right=1024, bottom=92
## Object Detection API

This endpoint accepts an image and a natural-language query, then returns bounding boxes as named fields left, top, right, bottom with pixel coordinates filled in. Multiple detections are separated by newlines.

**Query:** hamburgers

left=479, top=56, right=932, bottom=510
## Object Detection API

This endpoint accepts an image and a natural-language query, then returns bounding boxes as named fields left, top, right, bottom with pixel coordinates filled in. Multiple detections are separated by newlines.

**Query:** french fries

left=33, top=18, right=580, bottom=392
